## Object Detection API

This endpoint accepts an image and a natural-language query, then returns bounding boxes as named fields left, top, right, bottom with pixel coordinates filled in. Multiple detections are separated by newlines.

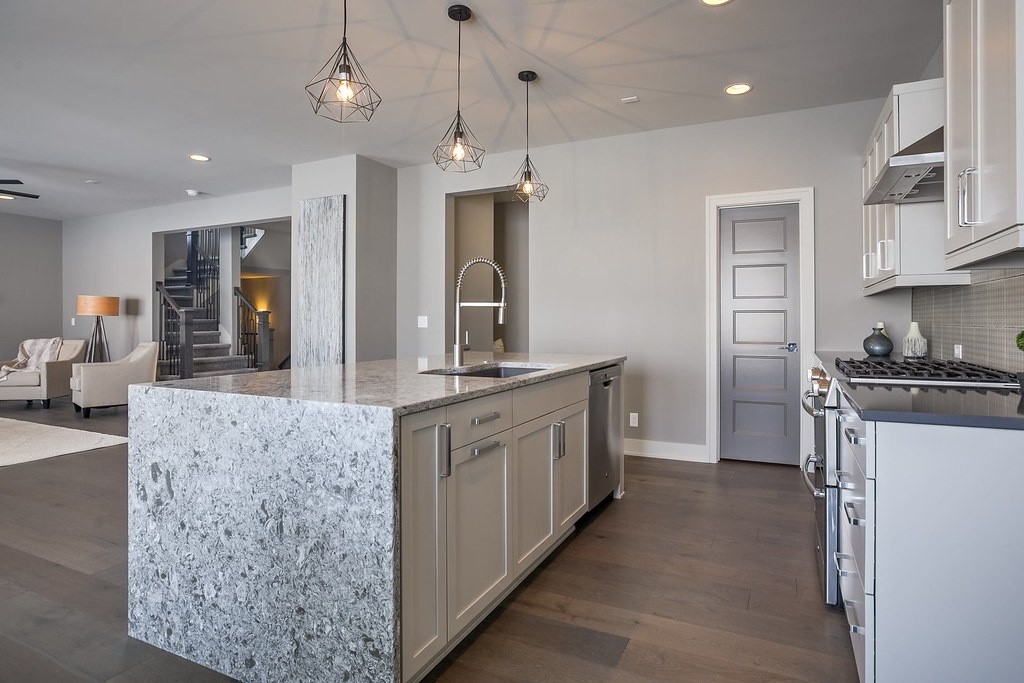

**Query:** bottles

left=864, top=327, right=893, bottom=355
left=903, top=322, right=927, bottom=357
left=876, top=322, right=890, bottom=338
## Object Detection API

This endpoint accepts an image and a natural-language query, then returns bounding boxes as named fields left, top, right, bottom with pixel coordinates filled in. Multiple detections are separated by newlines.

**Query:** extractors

left=863, top=126, right=944, bottom=205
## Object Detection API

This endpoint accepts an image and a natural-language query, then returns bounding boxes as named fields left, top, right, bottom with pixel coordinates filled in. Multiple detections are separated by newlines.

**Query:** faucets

left=453, top=256, right=508, bottom=367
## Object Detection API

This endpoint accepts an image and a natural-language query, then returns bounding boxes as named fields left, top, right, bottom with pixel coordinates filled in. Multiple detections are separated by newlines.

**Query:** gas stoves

left=807, top=357, right=1020, bottom=406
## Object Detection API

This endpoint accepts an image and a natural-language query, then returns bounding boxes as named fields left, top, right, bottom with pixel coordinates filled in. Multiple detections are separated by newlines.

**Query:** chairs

left=0, top=339, right=86, bottom=409
left=70, top=341, right=159, bottom=418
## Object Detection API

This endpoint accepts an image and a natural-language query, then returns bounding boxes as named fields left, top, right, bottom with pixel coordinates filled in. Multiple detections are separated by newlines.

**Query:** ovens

left=802, top=390, right=838, bottom=605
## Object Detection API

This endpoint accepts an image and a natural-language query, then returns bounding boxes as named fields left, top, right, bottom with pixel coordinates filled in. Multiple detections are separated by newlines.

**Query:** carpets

left=0, top=417, right=127, bottom=466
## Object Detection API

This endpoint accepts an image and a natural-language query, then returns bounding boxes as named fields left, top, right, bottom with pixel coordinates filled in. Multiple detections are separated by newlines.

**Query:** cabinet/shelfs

left=942, top=0, right=1024, bottom=269
left=862, top=77, right=971, bottom=297
left=834, top=391, right=1024, bottom=683
left=400, top=371, right=590, bottom=683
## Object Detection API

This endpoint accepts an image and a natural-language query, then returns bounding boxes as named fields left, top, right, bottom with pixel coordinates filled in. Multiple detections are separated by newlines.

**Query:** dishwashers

left=589, top=364, right=624, bottom=510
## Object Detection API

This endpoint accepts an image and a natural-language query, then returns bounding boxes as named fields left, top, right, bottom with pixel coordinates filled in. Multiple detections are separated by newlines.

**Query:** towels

left=0, top=337, right=64, bottom=383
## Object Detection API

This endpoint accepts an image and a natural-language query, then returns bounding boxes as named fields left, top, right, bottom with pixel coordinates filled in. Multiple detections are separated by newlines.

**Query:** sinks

left=416, top=360, right=568, bottom=378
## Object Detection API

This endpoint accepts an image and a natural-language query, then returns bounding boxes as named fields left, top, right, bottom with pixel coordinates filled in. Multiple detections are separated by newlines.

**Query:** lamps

left=305, top=0, right=382, bottom=123
left=432, top=5, right=486, bottom=173
left=77, top=295, right=120, bottom=363
left=506, top=71, right=549, bottom=203
